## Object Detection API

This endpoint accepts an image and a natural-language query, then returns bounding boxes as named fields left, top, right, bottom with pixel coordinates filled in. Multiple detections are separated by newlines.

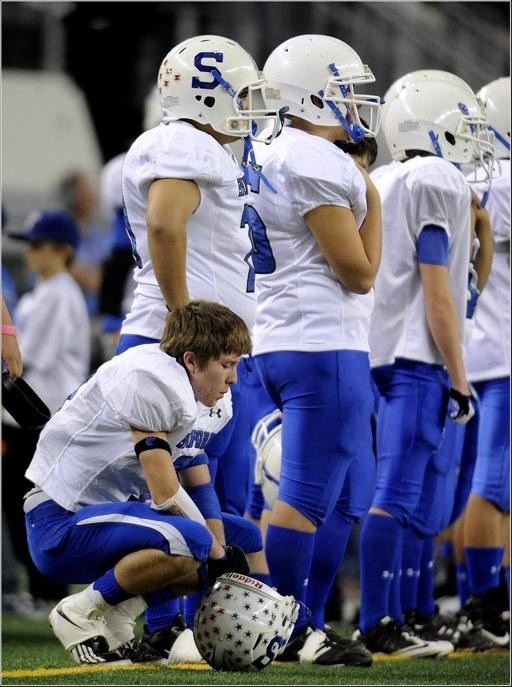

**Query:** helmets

left=155, top=34, right=290, bottom=146
left=475, top=76, right=512, bottom=160
left=260, top=32, right=385, bottom=142
left=189, top=572, right=300, bottom=672
left=250, top=407, right=283, bottom=508
left=377, top=67, right=504, bottom=184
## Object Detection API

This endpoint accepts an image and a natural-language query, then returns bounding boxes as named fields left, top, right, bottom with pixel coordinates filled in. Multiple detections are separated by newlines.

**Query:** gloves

left=208, top=545, right=251, bottom=588
left=446, top=387, right=476, bottom=426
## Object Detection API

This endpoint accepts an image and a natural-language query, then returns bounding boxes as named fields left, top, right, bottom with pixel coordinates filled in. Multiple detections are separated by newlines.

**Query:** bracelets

left=0, top=322, right=19, bottom=340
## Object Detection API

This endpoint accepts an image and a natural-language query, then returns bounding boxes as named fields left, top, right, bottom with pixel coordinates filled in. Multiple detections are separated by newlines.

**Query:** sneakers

left=413, top=616, right=491, bottom=653
left=394, top=623, right=452, bottom=661
left=105, top=606, right=167, bottom=667
left=349, top=616, right=441, bottom=658
left=274, top=627, right=373, bottom=671
left=47, top=594, right=131, bottom=670
left=139, top=613, right=208, bottom=666
left=453, top=610, right=511, bottom=649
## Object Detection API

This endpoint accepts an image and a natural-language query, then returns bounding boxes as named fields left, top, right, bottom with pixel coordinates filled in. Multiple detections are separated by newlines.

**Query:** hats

left=4, top=210, right=82, bottom=248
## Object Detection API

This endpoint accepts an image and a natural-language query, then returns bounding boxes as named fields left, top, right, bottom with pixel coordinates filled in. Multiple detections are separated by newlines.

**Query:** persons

left=454, top=75, right=512, bottom=651
left=59, top=169, right=124, bottom=360
left=244, top=116, right=379, bottom=591
left=0, top=292, right=29, bottom=385
left=352, top=68, right=502, bottom=662
left=242, top=32, right=383, bottom=668
left=24, top=298, right=264, bottom=669
left=117, top=35, right=282, bottom=668
left=399, top=186, right=492, bottom=646
left=3, top=206, right=91, bottom=430
